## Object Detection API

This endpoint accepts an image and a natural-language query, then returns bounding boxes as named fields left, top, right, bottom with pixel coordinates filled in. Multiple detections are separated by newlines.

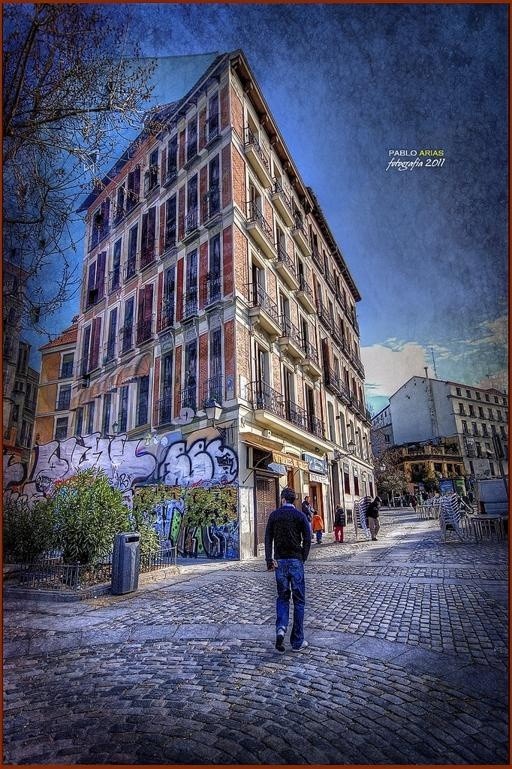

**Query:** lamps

left=336, top=440, right=357, bottom=459
left=204, top=398, right=237, bottom=430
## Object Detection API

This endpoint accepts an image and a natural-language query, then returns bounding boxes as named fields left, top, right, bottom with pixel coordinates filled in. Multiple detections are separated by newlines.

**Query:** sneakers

left=275, top=629, right=286, bottom=652
left=291, top=640, right=309, bottom=652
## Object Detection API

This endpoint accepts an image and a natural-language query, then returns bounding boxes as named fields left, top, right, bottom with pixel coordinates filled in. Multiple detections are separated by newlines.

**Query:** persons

left=334, top=504, right=345, bottom=544
left=366, top=496, right=383, bottom=541
left=302, top=494, right=315, bottom=523
left=264, top=487, right=312, bottom=652
left=405, top=491, right=430, bottom=513
left=311, top=510, right=323, bottom=545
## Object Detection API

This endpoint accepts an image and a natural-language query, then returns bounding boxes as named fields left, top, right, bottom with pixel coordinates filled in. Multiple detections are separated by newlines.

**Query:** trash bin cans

left=111, top=532, right=142, bottom=594
left=394, top=498, right=401, bottom=508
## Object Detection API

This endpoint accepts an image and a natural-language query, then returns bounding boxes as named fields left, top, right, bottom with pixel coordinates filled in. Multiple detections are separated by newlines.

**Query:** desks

left=470, top=512, right=504, bottom=544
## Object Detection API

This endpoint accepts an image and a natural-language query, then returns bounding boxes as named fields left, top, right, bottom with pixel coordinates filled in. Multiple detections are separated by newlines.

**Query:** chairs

left=434, top=489, right=470, bottom=544
left=350, top=496, right=372, bottom=540
